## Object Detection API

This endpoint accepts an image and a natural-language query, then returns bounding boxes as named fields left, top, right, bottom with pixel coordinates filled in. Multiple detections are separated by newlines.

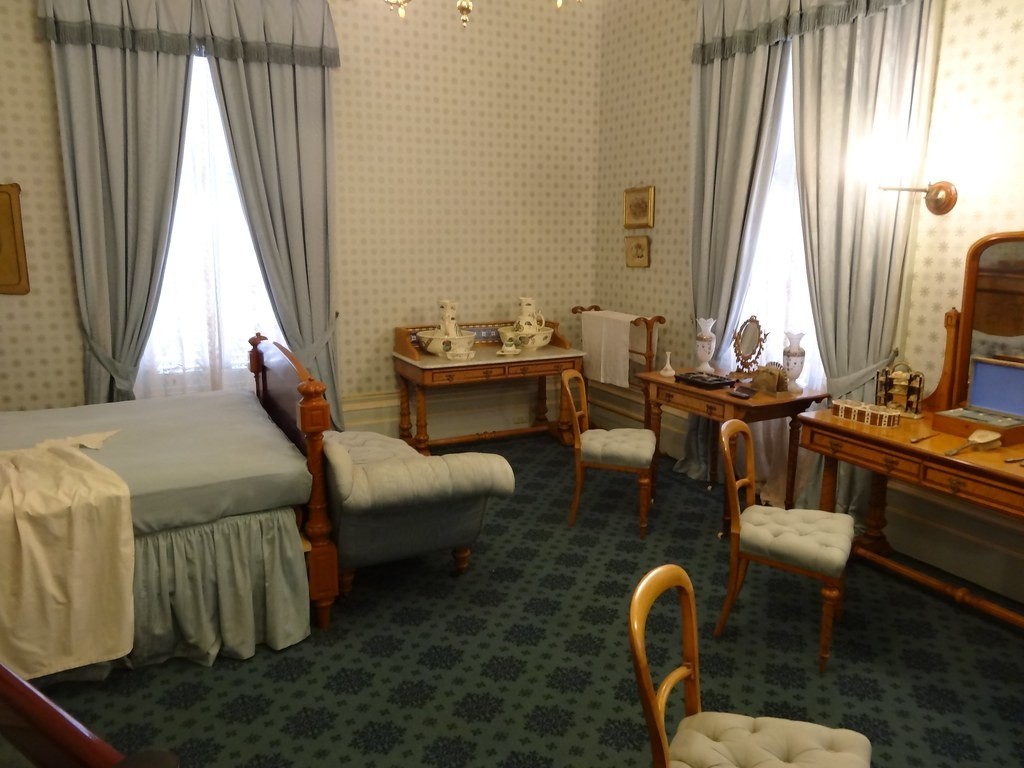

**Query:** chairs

left=714, top=419, right=856, bottom=671
left=629, top=564, right=871, bottom=768
left=560, top=367, right=657, bottom=537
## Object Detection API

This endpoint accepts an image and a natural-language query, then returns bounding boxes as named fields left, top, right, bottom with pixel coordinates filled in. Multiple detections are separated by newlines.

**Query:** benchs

left=325, top=428, right=514, bottom=595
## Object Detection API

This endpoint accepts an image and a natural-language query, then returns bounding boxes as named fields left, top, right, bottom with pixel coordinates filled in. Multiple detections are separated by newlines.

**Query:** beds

left=1, top=332, right=340, bottom=680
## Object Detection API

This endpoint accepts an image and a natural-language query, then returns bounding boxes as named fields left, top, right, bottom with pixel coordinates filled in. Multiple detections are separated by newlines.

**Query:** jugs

left=435, top=301, right=463, bottom=338
left=514, top=297, right=544, bottom=334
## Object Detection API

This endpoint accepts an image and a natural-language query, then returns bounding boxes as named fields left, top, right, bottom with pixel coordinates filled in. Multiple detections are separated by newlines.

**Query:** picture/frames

left=624, top=235, right=649, bottom=268
left=623, top=184, right=656, bottom=228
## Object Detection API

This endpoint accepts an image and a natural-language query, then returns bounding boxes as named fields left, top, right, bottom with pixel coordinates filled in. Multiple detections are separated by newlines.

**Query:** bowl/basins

left=498, top=326, right=554, bottom=350
left=447, top=351, right=476, bottom=362
left=417, top=329, right=477, bottom=355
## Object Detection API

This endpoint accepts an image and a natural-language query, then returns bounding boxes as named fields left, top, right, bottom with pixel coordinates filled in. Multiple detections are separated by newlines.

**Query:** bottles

left=501, top=334, right=516, bottom=351
left=660, top=352, right=675, bottom=377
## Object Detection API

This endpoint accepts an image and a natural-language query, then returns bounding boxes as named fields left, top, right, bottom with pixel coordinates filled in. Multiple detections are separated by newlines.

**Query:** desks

left=798, top=404, right=1024, bottom=627
left=638, top=370, right=831, bottom=539
left=394, top=318, right=587, bottom=455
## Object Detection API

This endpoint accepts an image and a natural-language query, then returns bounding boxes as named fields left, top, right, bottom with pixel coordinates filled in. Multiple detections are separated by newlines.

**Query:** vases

left=696, top=318, right=716, bottom=372
left=783, top=333, right=805, bottom=393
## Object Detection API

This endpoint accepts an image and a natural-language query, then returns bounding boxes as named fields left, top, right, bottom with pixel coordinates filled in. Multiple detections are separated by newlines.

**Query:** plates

left=495, top=349, right=523, bottom=356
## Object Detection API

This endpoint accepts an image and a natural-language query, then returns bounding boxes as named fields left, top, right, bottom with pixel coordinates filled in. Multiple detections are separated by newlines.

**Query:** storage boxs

left=932, top=356, right=1024, bottom=446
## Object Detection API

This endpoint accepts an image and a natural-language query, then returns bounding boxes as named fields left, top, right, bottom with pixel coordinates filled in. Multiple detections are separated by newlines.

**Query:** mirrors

left=923, top=232, right=1024, bottom=414
